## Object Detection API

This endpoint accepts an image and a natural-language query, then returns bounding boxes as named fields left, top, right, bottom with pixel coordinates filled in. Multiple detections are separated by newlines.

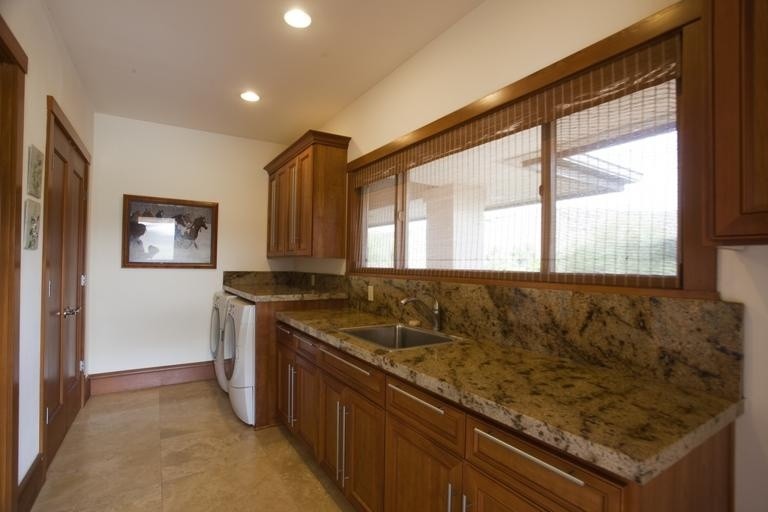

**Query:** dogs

left=130, top=208, right=208, bottom=251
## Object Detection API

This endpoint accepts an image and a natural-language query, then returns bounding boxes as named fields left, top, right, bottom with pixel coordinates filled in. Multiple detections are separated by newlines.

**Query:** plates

left=712, top=2, right=768, bottom=246
left=265, top=128, right=352, bottom=259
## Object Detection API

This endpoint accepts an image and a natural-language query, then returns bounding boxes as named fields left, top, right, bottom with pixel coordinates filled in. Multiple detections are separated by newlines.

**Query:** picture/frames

left=121, top=193, right=218, bottom=269
left=25, top=143, right=44, bottom=251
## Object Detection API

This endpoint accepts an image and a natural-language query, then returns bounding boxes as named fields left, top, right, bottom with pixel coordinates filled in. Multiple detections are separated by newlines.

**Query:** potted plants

left=25, top=143, right=44, bottom=251
left=121, top=193, right=218, bottom=269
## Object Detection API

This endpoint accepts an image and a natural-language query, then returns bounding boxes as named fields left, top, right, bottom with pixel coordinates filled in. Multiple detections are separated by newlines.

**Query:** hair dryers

left=209, top=292, right=237, bottom=393
left=222, top=299, right=255, bottom=426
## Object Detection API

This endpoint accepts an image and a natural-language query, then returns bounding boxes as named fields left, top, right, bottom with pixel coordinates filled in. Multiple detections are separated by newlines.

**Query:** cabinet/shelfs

left=317, top=345, right=385, bottom=510
left=278, top=324, right=322, bottom=464
left=265, top=128, right=352, bottom=259
left=385, top=381, right=734, bottom=511
left=712, top=2, right=768, bottom=246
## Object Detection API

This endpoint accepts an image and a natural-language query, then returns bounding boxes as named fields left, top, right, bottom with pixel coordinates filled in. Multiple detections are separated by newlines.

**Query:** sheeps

left=401, top=292, right=442, bottom=331
